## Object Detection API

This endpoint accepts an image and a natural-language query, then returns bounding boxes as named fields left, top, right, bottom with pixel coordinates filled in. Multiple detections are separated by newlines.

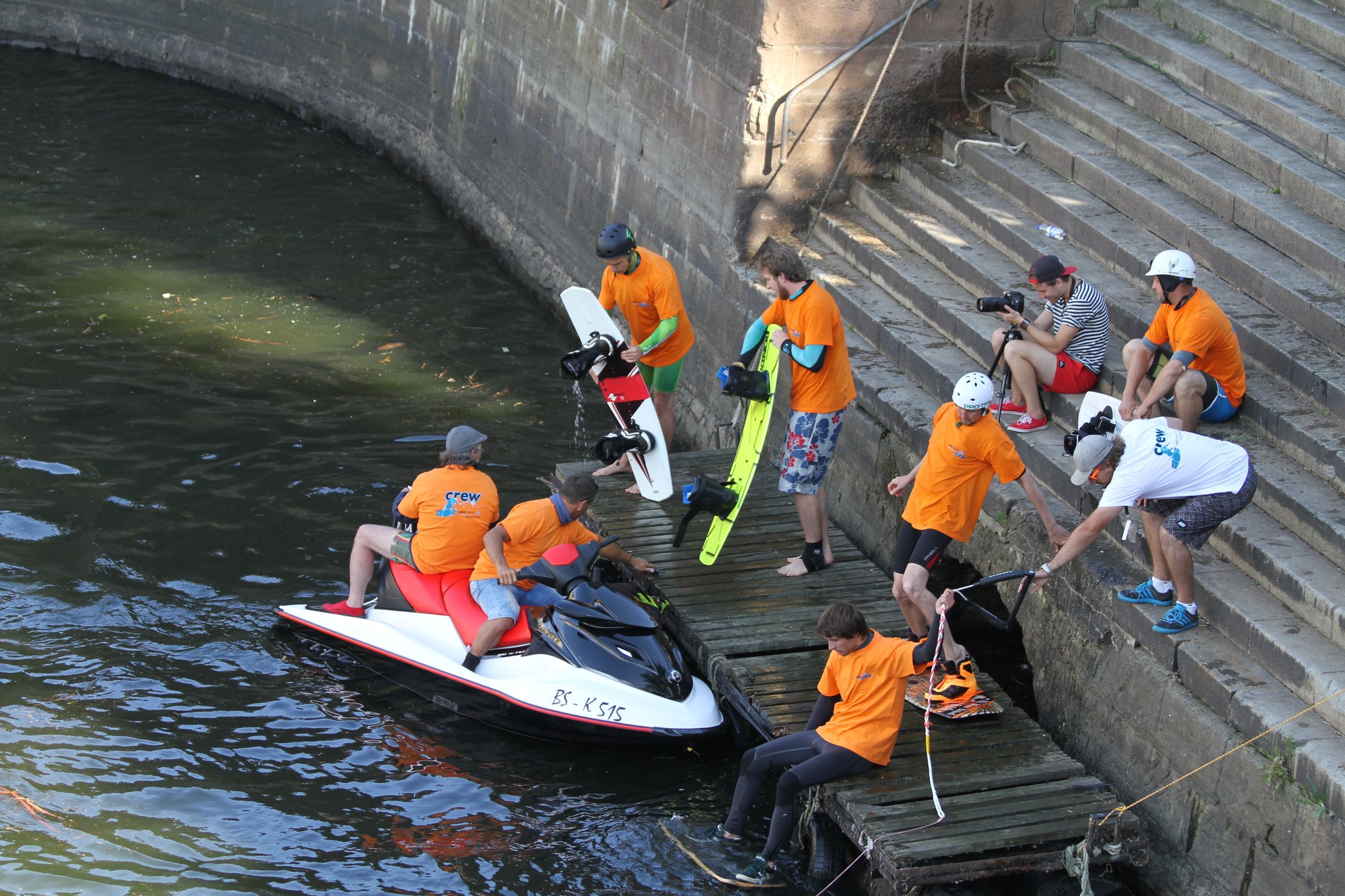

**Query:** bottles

left=1036, top=225, right=1065, bottom=240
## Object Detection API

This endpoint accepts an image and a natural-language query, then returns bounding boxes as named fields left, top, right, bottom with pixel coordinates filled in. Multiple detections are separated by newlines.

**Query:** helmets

left=952, top=372, right=993, bottom=410
left=596, top=223, right=637, bottom=259
left=1144, top=249, right=1197, bottom=279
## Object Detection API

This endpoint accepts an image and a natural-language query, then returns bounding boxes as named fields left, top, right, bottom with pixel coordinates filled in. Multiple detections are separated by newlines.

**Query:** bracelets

left=628, top=554, right=631, bottom=562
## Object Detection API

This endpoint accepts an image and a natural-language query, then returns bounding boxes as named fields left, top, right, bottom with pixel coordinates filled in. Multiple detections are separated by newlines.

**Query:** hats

left=1070, top=433, right=1113, bottom=486
left=1028, top=255, right=1078, bottom=284
left=445, top=425, right=488, bottom=454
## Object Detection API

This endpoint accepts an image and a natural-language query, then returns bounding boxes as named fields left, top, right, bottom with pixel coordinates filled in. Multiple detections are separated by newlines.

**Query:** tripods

left=988, top=313, right=1048, bottom=424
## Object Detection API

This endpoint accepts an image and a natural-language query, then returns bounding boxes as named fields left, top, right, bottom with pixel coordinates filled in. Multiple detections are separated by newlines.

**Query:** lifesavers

left=722, top=699, right=746, bottom=736
left=790, top=813, right=844, bottom=882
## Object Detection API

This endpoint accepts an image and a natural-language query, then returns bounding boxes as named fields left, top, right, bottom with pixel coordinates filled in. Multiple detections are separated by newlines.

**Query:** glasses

left=580, top=483, right=600, bottom=502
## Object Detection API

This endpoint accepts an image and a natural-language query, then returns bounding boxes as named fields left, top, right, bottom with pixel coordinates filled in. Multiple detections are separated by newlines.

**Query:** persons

left=320, top=425, right=500, bottom=619
left=593, top=224, right=696, bottom=494
left=719, top=588, right=956, bottom=882
left=1118, top=250, right=1246, bottom=433
left=1017, top=416, right=1257, bottom=634
left=462, top=473, right=655, bottom=672
left=888, top=373, right=1071, bottom=703
left=989, top=254, right=1109, bottom=432
left=733, top=247, right=857, bottom=577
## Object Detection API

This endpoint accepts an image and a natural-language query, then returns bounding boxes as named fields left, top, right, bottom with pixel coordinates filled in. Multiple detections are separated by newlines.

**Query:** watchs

left=1018, top=317, right=1030, bottom=331
left=1039, top=562, right=1054, bottom=578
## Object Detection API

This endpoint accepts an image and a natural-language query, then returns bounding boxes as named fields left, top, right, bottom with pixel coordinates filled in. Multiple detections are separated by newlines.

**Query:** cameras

left=1064, top=407, right=1116, bottom=456
left=977, top=291, right=1025, bottom=316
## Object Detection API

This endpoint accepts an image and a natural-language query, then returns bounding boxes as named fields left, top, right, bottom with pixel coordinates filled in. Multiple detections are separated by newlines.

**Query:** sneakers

left=1007, top=413, right=1049, bottom=433
left=590, top=429, right=655, bottom=467
left=1116, top=576, right=1175, bottom=606
left=558, top=334, right=617, bottom=381
left=716, top=364, right=768, bottom=403
left=736, top=854, right=782, bottom=883
left=322, top=599, right=367, bottom=619
left=906, top=626, right=940, bottom=669
left=1152, top=603, right=1198, bottom=633
left=988, top=396, right=1027, bottom=416
left=703, top=823, right=743, bottom=845
left=924, top=658, right=978, bottom=703
left=672, top=474, right=738, bottom=548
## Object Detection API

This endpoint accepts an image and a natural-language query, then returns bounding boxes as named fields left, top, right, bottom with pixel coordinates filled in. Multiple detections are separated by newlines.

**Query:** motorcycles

left=271, top=531, right=733, bottom=760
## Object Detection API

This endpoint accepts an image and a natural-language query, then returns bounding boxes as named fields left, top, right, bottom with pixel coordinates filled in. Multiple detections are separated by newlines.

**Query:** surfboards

left=658, top=817, right=785, bottom=889
left=558, top=285, right=674, bottom=502
left=698, top=324, right=782, bottom=567
